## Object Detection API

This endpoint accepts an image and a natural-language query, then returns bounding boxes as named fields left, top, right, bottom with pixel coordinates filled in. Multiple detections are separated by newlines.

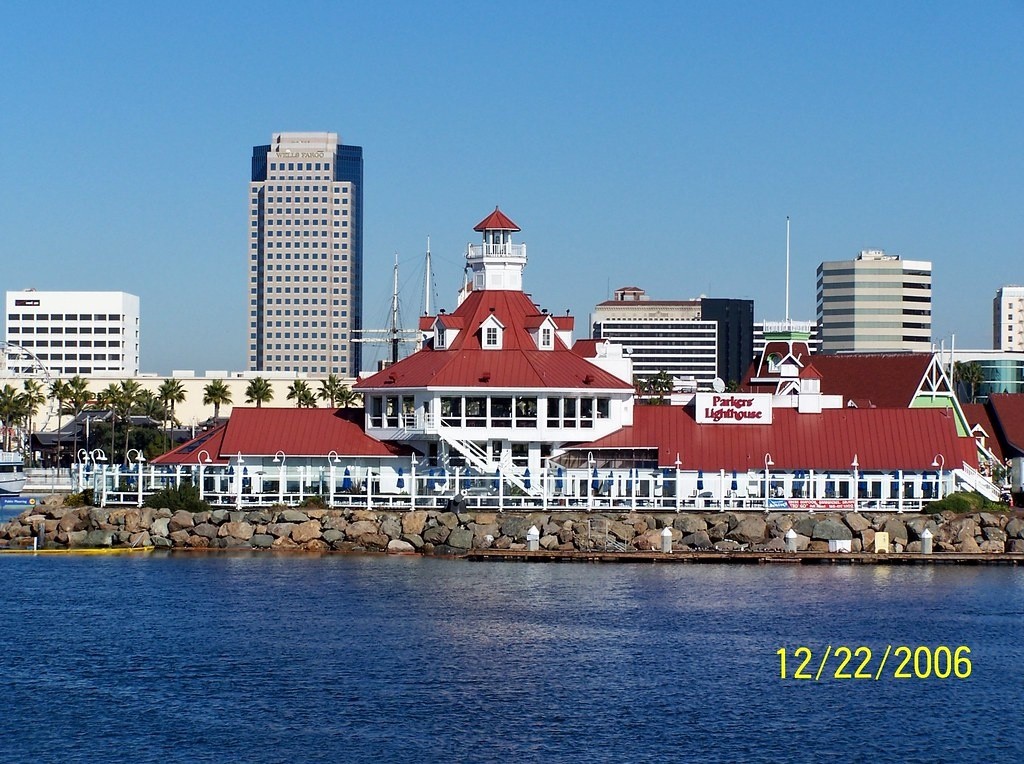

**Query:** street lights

left=235, top=449, right=245, bottom=512
left=273, top=450, right=286, bottom=506
left=851, top=453, right=859, bottom=514
left=197, top=448, right=212, bottom=501
left=674, top=452, right=682, bottom=513
left=931, top=453, right=945, bottom=500
left=328, top=451, right=341, bottom=509
left=586, top=451, right=596, bottom=508
left=76, top=448, right=146, bottom=509
left=409, top=452, right=419, bottom=511
left=764, top=452, right=775, bottom=514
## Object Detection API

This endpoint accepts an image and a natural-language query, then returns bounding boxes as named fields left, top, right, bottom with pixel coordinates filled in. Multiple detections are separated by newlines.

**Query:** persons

left=979, top=458, right=1012, bottom=484
left=999, top=493, right=1013, bottom=507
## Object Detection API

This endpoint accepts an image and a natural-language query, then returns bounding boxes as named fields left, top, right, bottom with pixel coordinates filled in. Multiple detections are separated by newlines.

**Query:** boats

left=0, top=448, right=28, bottom=498
left=1, top=543, right=156, bottom=556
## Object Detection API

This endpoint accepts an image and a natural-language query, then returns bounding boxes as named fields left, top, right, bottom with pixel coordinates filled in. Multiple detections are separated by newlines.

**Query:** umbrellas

left=342, top=466, right=931, bottom=500
